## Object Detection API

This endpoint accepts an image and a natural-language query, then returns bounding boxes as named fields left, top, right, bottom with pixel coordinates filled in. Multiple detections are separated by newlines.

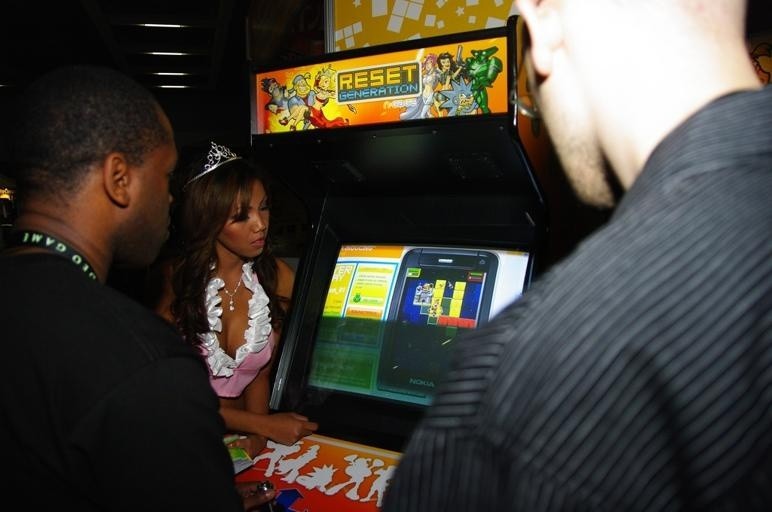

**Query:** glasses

left=506, top=52, right=538, bottom=119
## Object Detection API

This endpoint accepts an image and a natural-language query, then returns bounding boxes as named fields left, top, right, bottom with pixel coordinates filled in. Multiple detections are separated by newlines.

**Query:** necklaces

left=1, top=230, right=99, bottom=281
left=224, top=274, right=242, bottom=311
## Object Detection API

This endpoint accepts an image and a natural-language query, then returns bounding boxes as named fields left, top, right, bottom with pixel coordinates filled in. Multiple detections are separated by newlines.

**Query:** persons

left=158, top=141, right=317, bottom=459
left=0, top=66, right=277, bottom=512
left=382, top=0, right=772, bottom=512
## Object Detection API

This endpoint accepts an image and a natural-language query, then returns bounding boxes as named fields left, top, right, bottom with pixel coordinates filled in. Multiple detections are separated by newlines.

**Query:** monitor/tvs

left=284, top=226, right=541, bottom=435
